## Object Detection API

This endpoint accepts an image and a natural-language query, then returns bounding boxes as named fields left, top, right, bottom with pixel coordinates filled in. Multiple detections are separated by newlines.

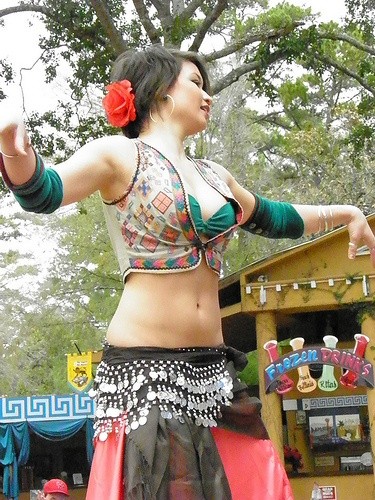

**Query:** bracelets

left=312, top=204, right=334, bottom=237
left=0, top=133, right=31, bottom=160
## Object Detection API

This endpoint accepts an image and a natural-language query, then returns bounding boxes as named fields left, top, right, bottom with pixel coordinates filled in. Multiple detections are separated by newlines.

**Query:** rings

left=349, top=242, right=358, bottom=249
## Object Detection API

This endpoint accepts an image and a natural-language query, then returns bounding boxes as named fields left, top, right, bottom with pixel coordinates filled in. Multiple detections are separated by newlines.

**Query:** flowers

left=102, top=79, right=137, bottom=128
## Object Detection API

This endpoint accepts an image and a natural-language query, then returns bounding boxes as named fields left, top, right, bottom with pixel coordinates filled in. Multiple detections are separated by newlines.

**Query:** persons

left=0, top=47, right=375, bottom=500
left=42, top=479, right=70, bottom=500
